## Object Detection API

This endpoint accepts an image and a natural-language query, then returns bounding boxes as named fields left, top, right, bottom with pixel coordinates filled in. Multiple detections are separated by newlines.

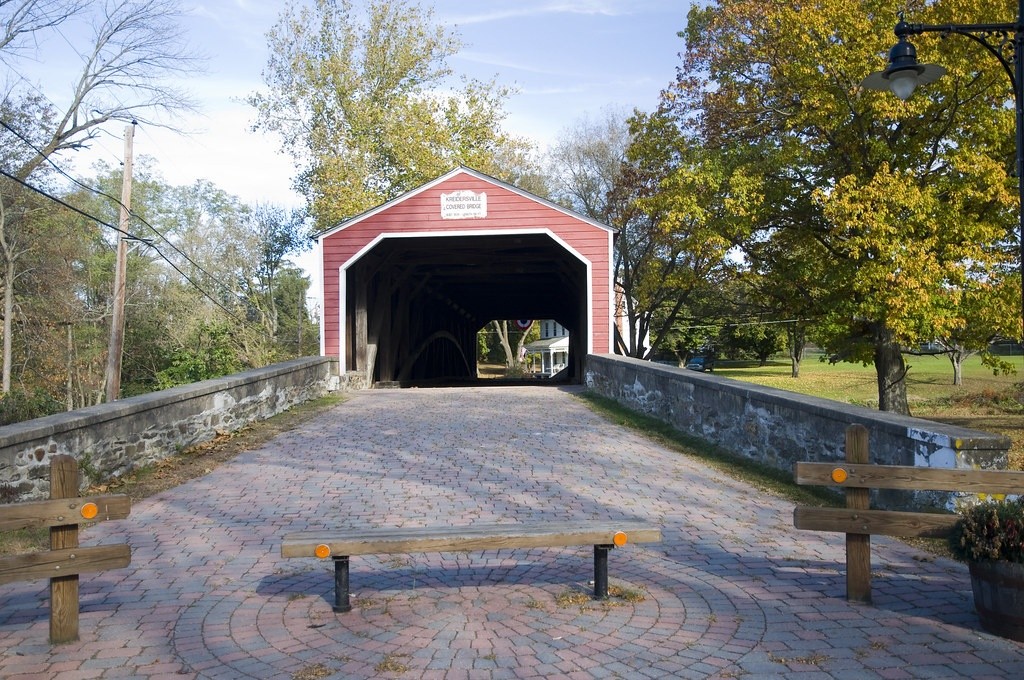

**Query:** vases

left=969, top=562, right=1024, bottom=645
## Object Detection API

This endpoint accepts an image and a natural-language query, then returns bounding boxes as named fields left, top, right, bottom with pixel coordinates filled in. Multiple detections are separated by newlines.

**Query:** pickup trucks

left=686, top=354, right=715, bottom=372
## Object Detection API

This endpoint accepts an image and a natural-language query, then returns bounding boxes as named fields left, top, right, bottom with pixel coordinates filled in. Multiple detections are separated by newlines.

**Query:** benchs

left=281, top=521, right=662, bottom=612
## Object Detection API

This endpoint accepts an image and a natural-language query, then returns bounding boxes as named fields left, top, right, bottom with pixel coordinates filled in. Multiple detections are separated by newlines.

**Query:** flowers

left=943, top=490, right=1024, bottom=564
